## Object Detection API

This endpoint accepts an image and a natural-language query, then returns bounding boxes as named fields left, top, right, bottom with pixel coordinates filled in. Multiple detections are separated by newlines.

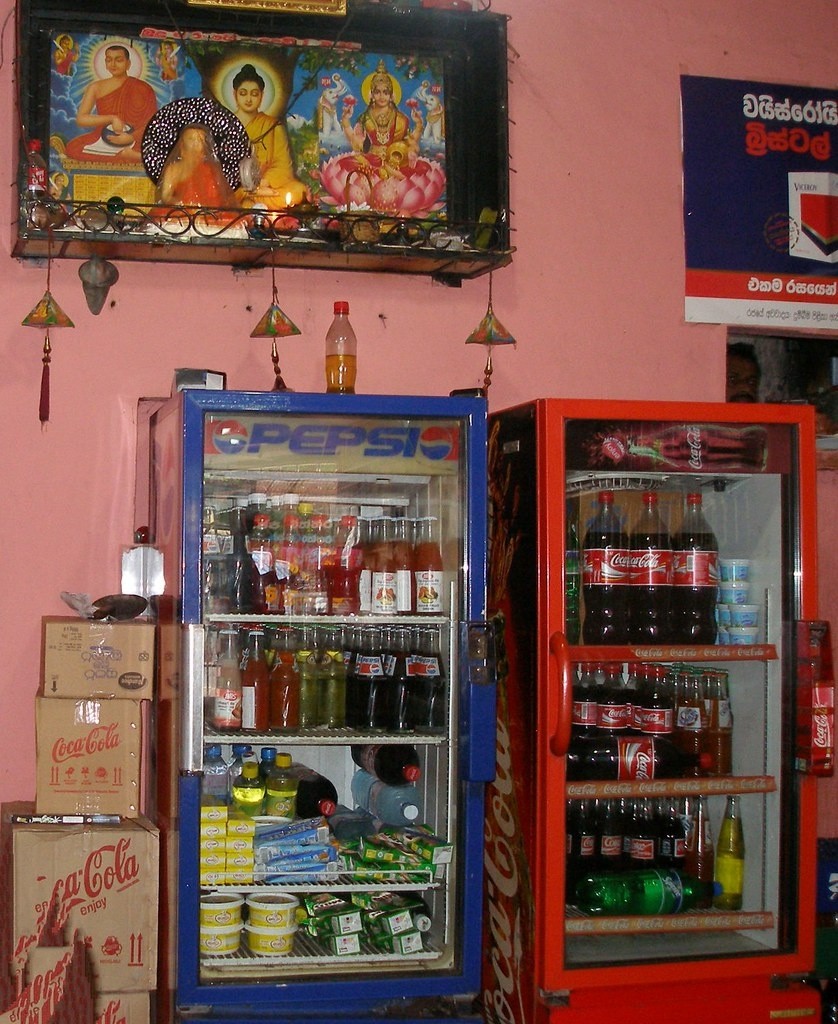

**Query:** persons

left=727, top=342, right=760, bottom=402
left=815, top=385, right=838, bottom=435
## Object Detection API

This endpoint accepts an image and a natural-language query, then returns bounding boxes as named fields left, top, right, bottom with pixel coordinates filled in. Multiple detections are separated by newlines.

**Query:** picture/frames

left=17, top=1, right=489, bottom=251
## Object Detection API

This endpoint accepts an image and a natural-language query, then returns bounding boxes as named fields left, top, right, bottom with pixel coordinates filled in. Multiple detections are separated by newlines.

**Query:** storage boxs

left=0, top=617, right=155, bottom=1024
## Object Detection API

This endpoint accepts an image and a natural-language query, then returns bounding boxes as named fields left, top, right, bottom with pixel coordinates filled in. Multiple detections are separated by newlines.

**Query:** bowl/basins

left=244, top=919, right=296, bottom=956
left=198, top=924, right=246, bottom=956
left=89, top=594, right=147, bottom=623
left=244, top=891, right=300, bottom=930
left=198, top=890, right=246, bottom=927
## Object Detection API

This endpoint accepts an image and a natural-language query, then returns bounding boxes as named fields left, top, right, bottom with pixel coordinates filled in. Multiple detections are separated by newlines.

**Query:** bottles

left=557, top=487, right=748, bottom=919
left=192, top=488, right=449, bottom=827
left=322, top=301, right=358, bottom=395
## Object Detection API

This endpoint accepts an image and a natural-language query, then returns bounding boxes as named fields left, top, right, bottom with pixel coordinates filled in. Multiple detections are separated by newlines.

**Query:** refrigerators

left=482, top=393, right=836, bottom=1021
left=150, top=389, right=496, bottom=1015
